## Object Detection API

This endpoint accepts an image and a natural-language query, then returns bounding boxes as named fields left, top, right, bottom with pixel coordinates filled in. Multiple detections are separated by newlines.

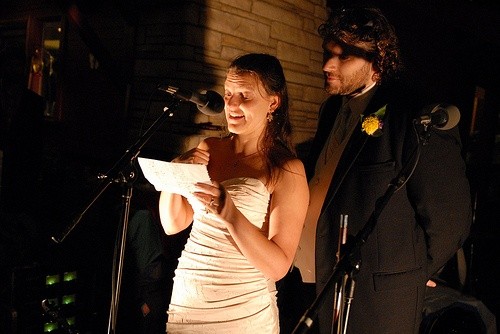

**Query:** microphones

left=151, top=80, right=225, bottom=116
left=412, top=102, right=460, bottom=130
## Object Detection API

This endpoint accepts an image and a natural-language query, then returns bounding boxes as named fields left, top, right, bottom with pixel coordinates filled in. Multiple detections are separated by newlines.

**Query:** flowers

left=359, top=107, right=386, bottom=137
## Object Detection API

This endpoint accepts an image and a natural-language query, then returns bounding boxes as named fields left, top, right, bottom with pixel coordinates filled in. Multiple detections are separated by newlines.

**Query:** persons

left=122, top=194, right=182, bottom=334
left=159, top=53, right=310, bottom=334
left=279, top=6, right=473, bottom=334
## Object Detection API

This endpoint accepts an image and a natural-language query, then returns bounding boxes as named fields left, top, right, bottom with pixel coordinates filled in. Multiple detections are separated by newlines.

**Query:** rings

left=209, top=196, right=215, bottom=206
